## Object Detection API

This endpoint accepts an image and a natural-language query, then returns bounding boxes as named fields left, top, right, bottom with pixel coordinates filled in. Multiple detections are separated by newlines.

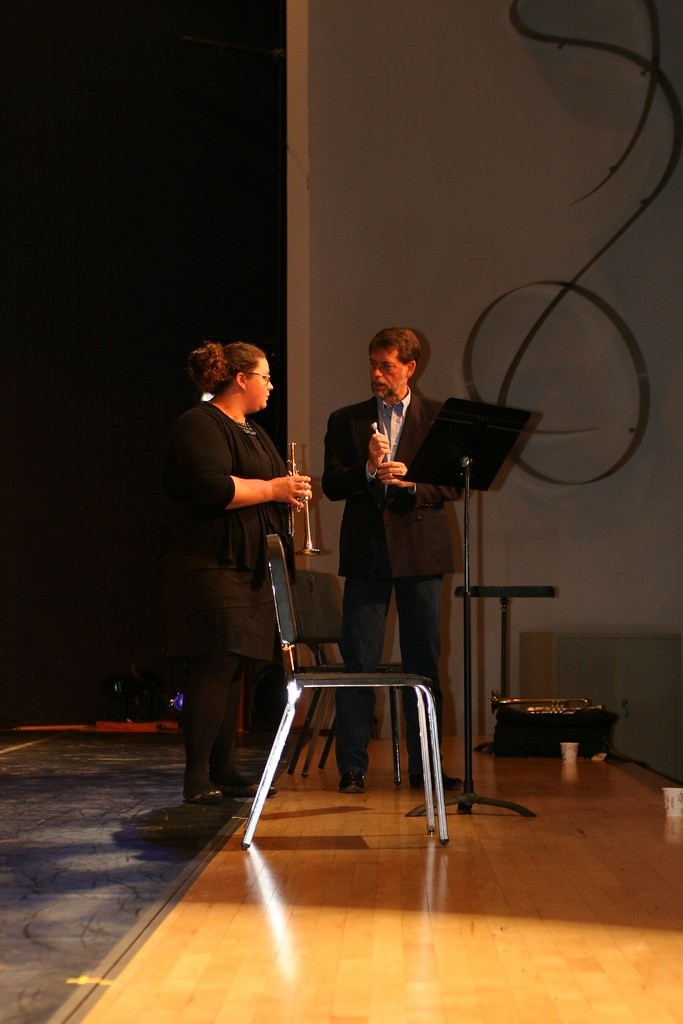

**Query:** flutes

left=371, top=422, right=391, bottom=463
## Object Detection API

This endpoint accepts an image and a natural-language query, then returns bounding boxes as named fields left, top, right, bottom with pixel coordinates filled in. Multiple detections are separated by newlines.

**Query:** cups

left=662, top=788, right=683, bottom=816
left=561, top=743, right=579, bottom=763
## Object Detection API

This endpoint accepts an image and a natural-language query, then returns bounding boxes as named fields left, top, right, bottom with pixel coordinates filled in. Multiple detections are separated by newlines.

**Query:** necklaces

left=233, top=420, right=257, bottom=435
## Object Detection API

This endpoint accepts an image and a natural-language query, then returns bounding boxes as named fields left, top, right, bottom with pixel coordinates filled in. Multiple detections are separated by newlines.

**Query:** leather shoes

left=338, top=771, right=366, bottom=795
left=408, top=769, right=463, bottom=790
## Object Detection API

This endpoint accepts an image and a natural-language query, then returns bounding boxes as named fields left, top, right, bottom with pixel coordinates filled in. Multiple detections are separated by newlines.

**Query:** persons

left=164, top=341, right=312, bottom=804
left=321, top=327, right=464, bottom=793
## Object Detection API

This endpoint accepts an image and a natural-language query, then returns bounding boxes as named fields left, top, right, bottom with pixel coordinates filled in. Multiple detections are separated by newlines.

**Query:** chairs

left=241, top=534, right=450, bottom=853
left=287, top=571, right=420, bottom=785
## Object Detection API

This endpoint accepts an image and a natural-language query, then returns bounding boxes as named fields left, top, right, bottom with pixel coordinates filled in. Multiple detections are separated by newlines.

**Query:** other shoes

left=222, top=783, right=278, bottom=798
left=180, top=787, right=227, bottom=804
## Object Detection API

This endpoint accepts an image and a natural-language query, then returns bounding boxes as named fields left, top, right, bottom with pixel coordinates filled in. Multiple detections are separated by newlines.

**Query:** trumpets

left=286, top=442, right=321, bottom=559
left=490, top=689, right=604, bottom=715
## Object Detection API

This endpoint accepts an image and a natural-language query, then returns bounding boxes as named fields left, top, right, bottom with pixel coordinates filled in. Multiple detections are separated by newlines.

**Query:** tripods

left=393, top=396, right=539, bottom=817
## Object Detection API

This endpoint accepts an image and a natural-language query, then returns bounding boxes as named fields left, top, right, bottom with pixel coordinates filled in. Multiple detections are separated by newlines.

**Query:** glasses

left=243, top=372, right=271, bottom=382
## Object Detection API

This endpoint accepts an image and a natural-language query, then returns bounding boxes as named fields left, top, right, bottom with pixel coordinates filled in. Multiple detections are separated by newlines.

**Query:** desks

left=455, top=585, right=557, bottom=756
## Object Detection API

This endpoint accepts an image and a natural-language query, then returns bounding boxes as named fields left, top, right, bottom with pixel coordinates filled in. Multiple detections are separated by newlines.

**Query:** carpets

left=0, top=736, right=294, bottom=1024
